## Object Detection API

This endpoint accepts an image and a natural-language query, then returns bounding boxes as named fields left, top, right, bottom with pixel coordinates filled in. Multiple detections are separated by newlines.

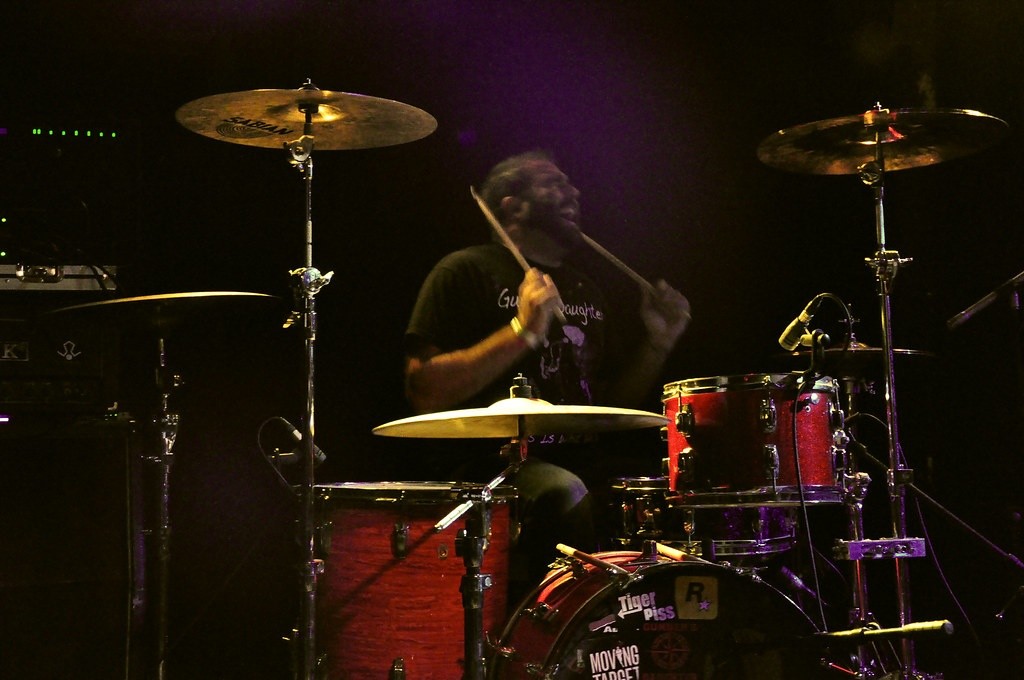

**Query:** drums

left=306, top=480, right=516, bottom=680
left=660, top=372, right=850, bottom=509
left=611, top=477, right=802, bottom=559
left=490, top=548, right=828, bottom=680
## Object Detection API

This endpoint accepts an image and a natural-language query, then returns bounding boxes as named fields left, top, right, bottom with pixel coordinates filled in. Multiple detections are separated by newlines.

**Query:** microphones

left=779, top=295, right=823, bottom=351
left=280, top=417, right=326, bottom=464
left=711, top=631, right=742, bottom=680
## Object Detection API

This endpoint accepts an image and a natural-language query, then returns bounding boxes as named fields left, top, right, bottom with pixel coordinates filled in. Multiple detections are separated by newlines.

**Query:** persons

left=403, top=153, right=691, bottom=596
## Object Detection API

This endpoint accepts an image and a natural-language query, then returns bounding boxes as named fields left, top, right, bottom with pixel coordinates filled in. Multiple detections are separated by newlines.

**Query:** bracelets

left=509, top=317, right=540, bottom=350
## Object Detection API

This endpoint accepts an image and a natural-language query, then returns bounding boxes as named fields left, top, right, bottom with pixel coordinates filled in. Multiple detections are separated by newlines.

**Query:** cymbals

left=374, top=399, right=671, bottom=442
left=176, top=88, right=438, bottom=150
left=758, top=110, right=1010, bottom=178
left=791, top=336, right=930, bottom=356
left=50, top=291, right=279, bottom=322
